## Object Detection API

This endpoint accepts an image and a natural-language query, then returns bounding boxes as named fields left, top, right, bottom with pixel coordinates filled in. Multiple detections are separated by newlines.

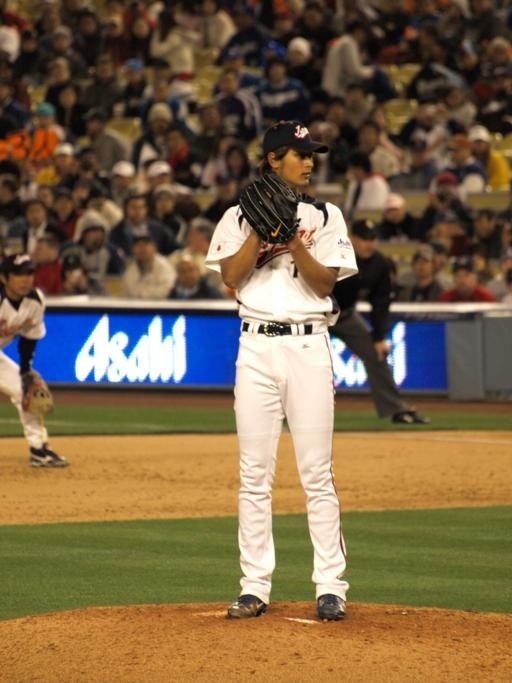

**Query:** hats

left=263, top=120, right=328, bottom=156
left=0, top=103, right=177, bottom=274
left=342, top=123, right=492, bottom=273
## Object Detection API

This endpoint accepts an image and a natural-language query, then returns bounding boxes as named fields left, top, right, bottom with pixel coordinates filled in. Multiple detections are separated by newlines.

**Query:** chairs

left=22, top=53, right=512, bottom=296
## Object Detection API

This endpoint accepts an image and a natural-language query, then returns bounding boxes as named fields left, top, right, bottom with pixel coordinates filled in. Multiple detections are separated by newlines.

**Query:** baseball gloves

left=19, top=369, right=55, bottom=425
left=239, top=175, right=300, bottom=245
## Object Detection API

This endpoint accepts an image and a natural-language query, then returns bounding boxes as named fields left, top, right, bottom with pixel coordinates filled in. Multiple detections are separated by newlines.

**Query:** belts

left=242, top=322, right=312, bottom=337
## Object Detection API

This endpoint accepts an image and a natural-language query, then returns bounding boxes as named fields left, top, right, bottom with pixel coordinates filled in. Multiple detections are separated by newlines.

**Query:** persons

left=203, top=121, right=360, bottom=625
left=0, top=254, right=68, bottom=469
left=328, top=219, right=432, bottom=427
left=0, top=0, right=512, bottom=303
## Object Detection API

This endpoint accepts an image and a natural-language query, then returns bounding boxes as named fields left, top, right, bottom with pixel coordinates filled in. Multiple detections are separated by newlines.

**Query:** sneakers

left=392, top=411, right=430, bottom=424
left=317, top=593, right=347, bottom=620
left=29, top=442, right=69, bottom=467
left=227, top=594, right=267, bottom=619
